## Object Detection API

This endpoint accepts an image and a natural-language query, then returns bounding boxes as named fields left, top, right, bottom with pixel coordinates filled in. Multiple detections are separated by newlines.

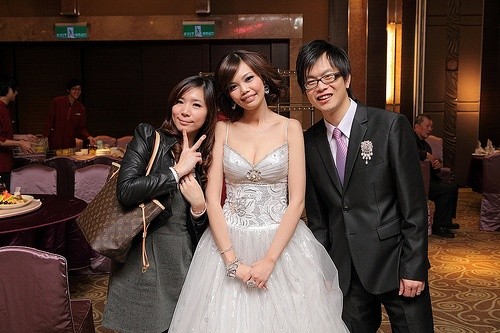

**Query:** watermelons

left=2, top=190, right=13, bottom=202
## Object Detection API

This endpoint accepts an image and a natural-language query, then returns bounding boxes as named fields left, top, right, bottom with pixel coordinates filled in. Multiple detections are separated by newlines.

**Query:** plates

left=0, top=195, right=34, bottom=209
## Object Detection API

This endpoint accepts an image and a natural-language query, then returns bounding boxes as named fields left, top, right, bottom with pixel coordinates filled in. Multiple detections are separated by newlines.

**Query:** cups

left=81, top=149, right=88, bottom=155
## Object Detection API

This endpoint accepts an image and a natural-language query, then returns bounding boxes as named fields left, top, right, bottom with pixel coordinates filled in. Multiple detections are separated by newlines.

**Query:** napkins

left=485, top=139, right=496, bottom=153
left=475, top=140, right=485, bottom=154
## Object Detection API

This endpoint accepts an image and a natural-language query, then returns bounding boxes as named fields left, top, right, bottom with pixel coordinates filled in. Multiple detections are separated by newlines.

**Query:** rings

left=247, top=280, right=256, bottom=287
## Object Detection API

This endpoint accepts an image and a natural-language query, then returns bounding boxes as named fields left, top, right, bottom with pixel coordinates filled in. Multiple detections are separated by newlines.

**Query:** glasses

left=71, top=88, right=81, bottom=91
left=303, top=72, right=341, bottom=90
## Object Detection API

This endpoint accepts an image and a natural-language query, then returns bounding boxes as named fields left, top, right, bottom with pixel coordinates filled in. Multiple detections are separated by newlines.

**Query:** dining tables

left=470, top=149, right=500, bottom=232
left=0, top=193, right=87, bottom=248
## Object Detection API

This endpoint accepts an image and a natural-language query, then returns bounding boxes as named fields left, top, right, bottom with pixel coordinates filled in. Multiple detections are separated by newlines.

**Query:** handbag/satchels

left=75, top=130, right=166, bottom=264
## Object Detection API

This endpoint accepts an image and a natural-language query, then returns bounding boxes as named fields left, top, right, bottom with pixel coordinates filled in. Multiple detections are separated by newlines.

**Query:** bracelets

left=226, top=258, right=240, bottom=278
left=221, top=247, right=232, bottom=254
left=193, top=207, right=205, bottom=214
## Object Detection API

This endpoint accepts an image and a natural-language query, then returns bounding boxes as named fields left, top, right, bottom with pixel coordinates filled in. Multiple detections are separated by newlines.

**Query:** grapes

left=0, top=201, right=12, bottom=204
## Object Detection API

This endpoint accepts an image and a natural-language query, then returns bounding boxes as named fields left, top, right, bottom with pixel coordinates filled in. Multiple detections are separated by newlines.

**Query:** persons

left=0, top=78, right=38, bottom=188
left=43, top=82, right=96, bottom=149
left=413, top=114, right=460, bottom=239
left=103, top=76, right=215, bottom=333
left=296, top=40, right=435, bottom=333
left=168, top=49, right=351, bottom=333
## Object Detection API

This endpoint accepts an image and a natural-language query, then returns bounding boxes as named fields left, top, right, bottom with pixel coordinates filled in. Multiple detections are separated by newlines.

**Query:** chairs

left=11, top=136, right=132, bottom=273
left=420, top=136, right=443, bottom=237
left=0, top=245, right=95, bottom=333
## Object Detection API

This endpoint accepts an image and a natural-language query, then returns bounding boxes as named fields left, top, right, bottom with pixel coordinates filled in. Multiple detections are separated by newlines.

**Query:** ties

left=333, top=129, right=348, bottom=187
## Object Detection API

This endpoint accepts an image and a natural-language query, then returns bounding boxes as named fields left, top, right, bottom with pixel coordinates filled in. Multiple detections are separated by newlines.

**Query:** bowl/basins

left=75, top=152, right=83, bottom=156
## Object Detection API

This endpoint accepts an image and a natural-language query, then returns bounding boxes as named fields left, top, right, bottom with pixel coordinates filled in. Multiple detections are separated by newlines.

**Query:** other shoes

left=446, top=223, right=460, bottom=229
left=432, top=226, right=452, bottom=237
